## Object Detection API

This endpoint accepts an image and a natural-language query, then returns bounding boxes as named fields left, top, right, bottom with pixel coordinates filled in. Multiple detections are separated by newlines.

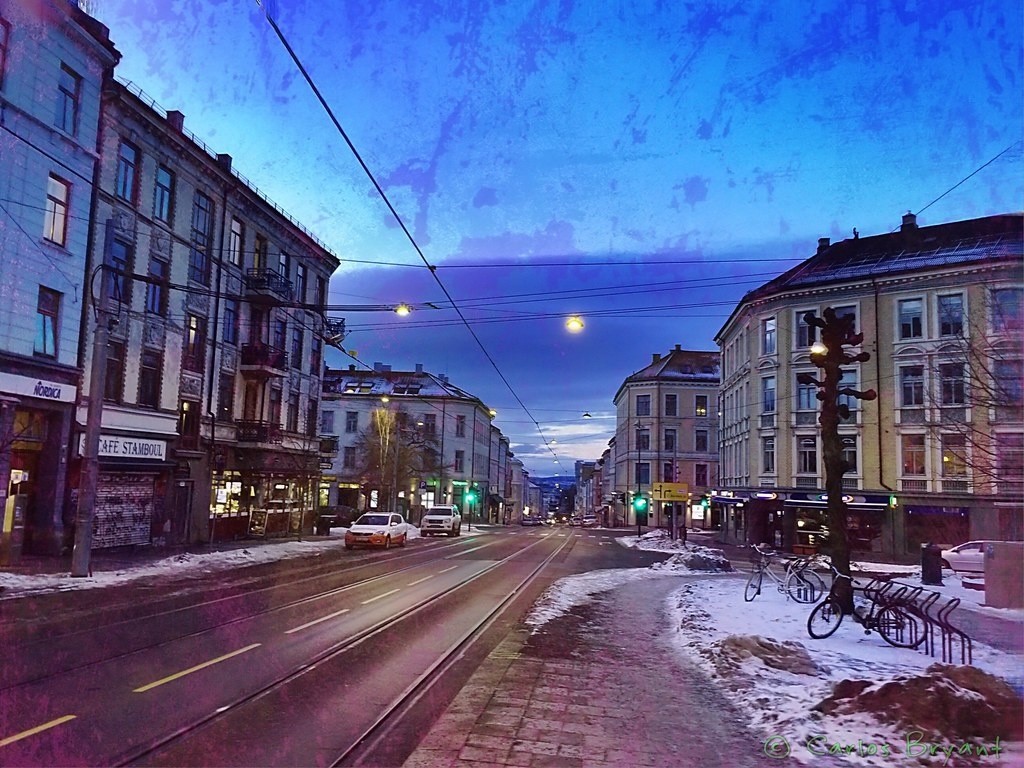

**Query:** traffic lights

left=636, top=496, right=644, bottom=511
left=701, top=497, right=708, bottom=507
left=468, top=490, right=474, bottom=504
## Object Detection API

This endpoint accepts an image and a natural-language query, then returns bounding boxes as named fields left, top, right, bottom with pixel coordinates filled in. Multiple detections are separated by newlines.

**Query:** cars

left=580, top=514, right=598, bottom=527
left=571, top=517, right=583, bottom=526
left=534, top=517, right=556, bottom=525
left=522, top=517, right=534, bottom=526
left=940, top=540, right=987, bottom=572
left=345, top=510, right=409, bottom=549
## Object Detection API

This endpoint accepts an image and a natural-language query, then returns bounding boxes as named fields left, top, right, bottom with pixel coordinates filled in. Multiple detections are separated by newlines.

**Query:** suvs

left=420, top=503, right=461, bottom=537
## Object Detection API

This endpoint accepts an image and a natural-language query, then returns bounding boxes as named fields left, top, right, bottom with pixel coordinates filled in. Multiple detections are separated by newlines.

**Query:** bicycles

left=806, top=558, right=930, bottom=649
left=744, top=541, right=826, bottom=604
